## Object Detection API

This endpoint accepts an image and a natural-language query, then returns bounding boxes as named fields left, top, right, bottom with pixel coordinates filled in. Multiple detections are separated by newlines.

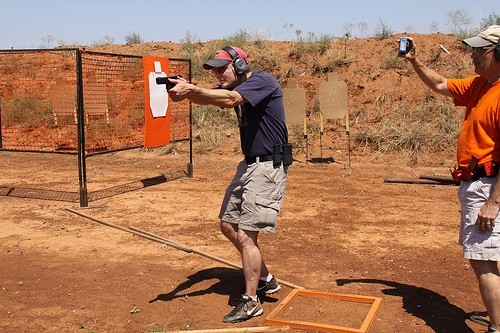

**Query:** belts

left=472, top=167, right=498, bottom=182
left=245, top=155, right=274, bottom=164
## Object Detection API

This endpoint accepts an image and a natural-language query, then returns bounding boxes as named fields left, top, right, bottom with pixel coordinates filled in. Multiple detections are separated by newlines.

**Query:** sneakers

left=241, top=275, right=281, bottom=295
left=222, top=294, right=264, bottom=324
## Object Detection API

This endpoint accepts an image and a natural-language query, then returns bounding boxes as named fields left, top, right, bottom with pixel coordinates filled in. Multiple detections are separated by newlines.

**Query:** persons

left=397, top=25, right=500, bottom=333
left=167, top=45, right=289, bottom=322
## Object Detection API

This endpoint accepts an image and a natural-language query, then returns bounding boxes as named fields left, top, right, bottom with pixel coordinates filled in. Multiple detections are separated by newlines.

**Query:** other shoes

left=470, top=310, right=499, bottom=333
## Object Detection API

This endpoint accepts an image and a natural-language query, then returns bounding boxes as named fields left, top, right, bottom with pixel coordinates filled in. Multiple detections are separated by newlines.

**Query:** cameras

left=399, top=38, right=412, bottom=53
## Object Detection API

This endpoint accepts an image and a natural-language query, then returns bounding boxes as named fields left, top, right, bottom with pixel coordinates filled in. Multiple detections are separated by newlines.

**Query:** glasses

left=472, top=47, right=492, bottom=55
left=210, top=67, right=230, bottom=74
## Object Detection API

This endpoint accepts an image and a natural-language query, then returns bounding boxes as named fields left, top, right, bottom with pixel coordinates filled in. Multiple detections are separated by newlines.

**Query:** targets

left=282, top=79, right=309, bottom=165
left=318, top=72, right=350, bottom=167
left=148, top=61, right=169, bottom=118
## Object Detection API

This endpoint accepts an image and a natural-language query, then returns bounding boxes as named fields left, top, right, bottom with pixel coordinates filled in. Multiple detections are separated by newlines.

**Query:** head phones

left=495, top=38, right=500, bottom=61
left=223, top=46, right=249, bottom=75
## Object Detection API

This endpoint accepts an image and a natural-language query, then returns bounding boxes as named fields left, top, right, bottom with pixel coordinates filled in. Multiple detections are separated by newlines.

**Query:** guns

left=156, top=74, right=183, bottom=92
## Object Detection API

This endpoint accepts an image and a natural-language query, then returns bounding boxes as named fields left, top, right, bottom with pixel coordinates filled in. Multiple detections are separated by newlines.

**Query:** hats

left=202, top=45, right=249, bottom=70
left=461, top=24, right=500, bottom=48
